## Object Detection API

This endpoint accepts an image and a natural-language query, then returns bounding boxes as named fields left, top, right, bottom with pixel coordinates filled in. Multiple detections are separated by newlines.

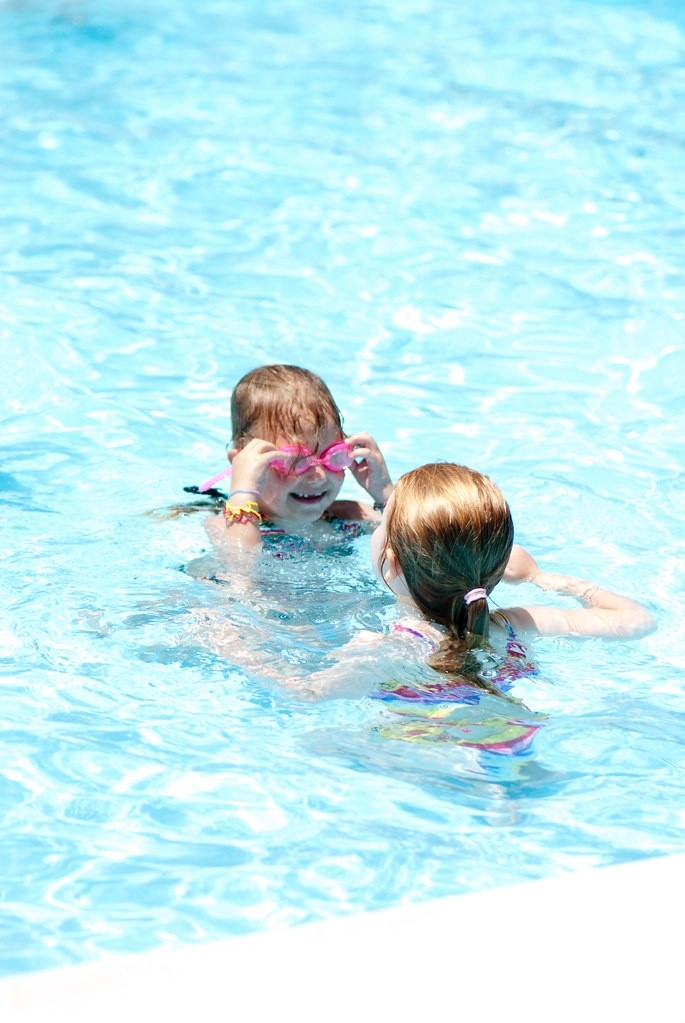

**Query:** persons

left=201, top=364, right=395, bottom=539
left=370, top=462, right=538, bottom=642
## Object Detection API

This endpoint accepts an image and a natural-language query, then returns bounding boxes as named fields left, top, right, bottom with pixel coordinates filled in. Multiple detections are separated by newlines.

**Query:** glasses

left=199, top=440, right=354, bottom=493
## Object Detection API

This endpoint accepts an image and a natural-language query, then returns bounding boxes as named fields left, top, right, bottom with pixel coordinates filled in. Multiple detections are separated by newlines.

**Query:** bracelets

left=223, top=489, right=263, bottom=525
left=373, top=502, right=386, bottom=511
